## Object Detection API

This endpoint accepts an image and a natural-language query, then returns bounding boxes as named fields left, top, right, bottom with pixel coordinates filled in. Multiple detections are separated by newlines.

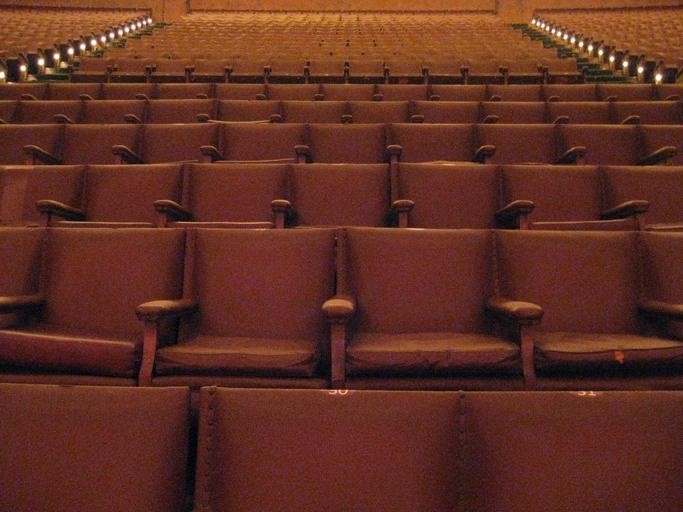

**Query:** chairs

left=11, top=124, right=670, bottom=166
left=0, top=5, right=152, bottom=83
left=74, top=12, right=589, bottom=83
left=11, top=163, right=669, bottom=231
left=532, top=7, right=683, bottom=85
left=1, top=226, right=658, bottom=391
left=1, top=385, right=664, bottom=512
left=11, top=82, right=670, bottom=104
left=11, top=100, right=670, bottom=125
